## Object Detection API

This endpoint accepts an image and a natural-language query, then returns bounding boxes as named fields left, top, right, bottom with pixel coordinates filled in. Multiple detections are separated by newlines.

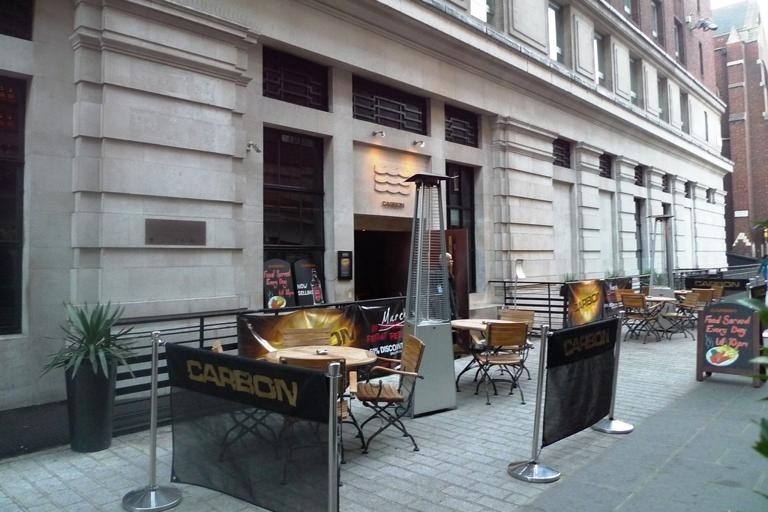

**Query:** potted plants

left=39, top=301, right=141, bottom=453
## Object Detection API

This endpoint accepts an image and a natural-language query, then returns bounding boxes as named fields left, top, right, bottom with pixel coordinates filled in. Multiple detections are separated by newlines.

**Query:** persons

left=756, top=254, right=768, bottom=280
left=430, top=252, right=458, bottom=321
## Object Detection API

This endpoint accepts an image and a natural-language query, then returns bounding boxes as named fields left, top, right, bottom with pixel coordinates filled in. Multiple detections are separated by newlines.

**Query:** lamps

left=247, top=141, right=262, bottom=153
left=372, top=130, right=385, bottom=137
left=691, top=17, right=718, bottom=31
left=414, top=140, right=425, bottom=147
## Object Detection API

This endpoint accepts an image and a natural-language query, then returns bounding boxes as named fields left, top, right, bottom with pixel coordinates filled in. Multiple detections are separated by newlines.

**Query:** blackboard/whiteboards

left=264, top=258, right=296, bottom=308
left=294, top=258, right=325, bottom=306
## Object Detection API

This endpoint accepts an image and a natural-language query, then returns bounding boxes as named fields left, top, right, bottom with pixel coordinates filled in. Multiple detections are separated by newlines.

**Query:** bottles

left=310, top=268, right=324, bottom=304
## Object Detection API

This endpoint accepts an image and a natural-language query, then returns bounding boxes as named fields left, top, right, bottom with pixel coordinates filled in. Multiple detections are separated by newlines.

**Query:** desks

left=258, top=346, right=378, bottom=449
left=451, top=319, right=513, bottom=396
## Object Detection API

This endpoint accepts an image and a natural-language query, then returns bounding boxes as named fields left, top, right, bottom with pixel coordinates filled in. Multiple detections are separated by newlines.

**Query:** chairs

left=476, top=322, right=528, bottom=405
left=280, top=357, right=351, bottom=486
left=614, top=286, right=724, bottom=343
left=356, top=335, right=424, bottom=454
left=212, top=340, right=281, bottom=463
left=498, top=310, right=534, bottom=380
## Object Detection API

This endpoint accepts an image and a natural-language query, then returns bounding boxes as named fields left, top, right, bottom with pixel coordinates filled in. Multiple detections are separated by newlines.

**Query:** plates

left=706, top=347, right=739, bottom=367
left=267, top=295, right=287, bottom=309
